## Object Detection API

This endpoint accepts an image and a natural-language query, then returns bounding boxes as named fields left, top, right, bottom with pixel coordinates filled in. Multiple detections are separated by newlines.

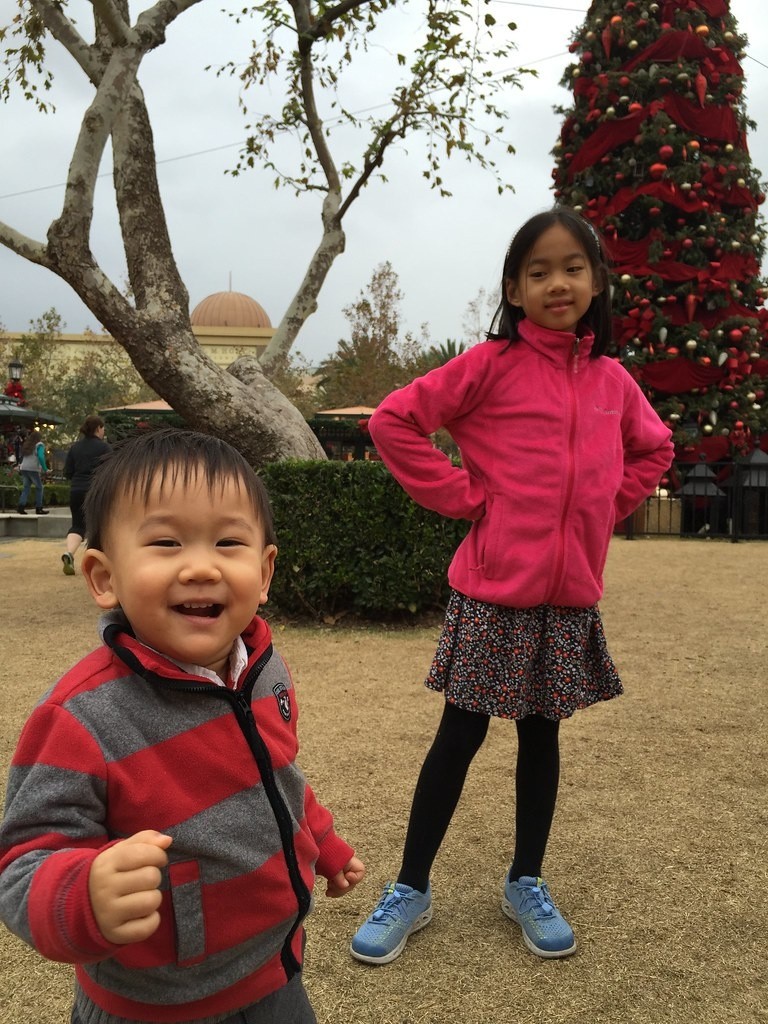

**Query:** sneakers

left=62, top=552, right=75, bottom=576
left=501, top=864, right=577, bottom=957
left=349, top=881, right=433, bottom=964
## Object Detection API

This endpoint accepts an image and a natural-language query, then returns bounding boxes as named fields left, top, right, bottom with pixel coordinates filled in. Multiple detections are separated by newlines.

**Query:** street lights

left=6, top=351, right=27, bottom=466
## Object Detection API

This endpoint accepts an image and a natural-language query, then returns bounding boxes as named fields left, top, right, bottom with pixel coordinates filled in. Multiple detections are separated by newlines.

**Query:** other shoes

left=17, top=505, right=28, bottom=514
left=36, top=506, right=49, bottom=514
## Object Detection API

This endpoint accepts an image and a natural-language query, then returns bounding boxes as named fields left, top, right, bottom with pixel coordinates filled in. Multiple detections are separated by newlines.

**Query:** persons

left=349, top=209, right=674, bottom=965
left=0, top=428, right=366, bottom=1024
left=16, top=415, right=115, bottom=574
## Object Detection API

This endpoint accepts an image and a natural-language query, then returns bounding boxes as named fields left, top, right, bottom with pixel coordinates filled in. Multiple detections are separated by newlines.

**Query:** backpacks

left=22, top=441, right=34, bottom=455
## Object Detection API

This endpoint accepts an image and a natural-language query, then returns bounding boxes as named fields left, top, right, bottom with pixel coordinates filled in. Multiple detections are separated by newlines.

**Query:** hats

left=29, top=431, right=42, bottom=441
